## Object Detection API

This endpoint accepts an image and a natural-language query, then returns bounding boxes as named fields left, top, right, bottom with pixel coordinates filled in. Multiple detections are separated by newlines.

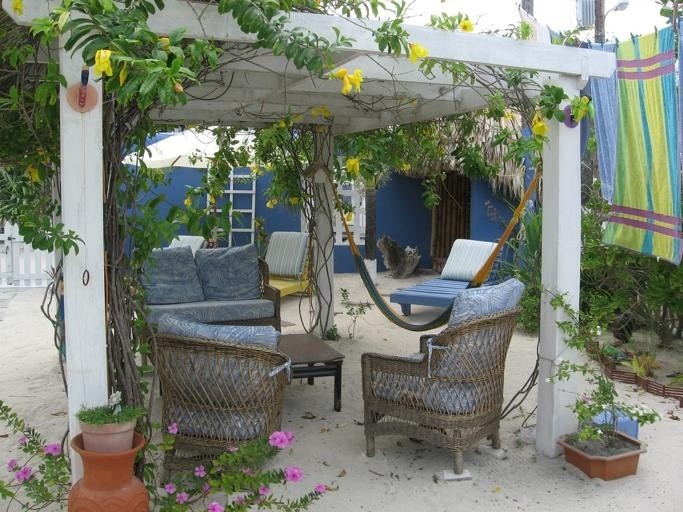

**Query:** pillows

left=194, top=243, right=262, bottom=300
left=435, top=278, right=525, bottom=379
left=156, top=312, right=279, bottom=405
left=139, top=246, right=206, bottom=304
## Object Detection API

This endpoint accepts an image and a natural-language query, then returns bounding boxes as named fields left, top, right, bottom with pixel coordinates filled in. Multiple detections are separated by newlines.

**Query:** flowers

left=532, top=277, right=661, bottom=450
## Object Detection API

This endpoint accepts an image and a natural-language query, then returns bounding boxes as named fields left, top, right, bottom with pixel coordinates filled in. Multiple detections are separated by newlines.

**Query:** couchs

left=128, top=258, right=281, bottom=371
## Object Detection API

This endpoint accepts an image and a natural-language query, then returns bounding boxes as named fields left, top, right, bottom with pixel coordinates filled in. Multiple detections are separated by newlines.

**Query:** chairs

left=146, top=334, right=291, bottom=487
left=389, top=238, right=499, bottom=316
left=168, top=234, right=208, bottom=261
left=361, top=305, right=523, bottom=473
left=256, top=231, right=314, bottom=298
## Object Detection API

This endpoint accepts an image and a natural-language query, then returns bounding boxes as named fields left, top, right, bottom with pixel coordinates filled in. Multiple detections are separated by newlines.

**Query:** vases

left=558, top=428, right=649, bottom=481
left=68, top=432, right=149, bottom=512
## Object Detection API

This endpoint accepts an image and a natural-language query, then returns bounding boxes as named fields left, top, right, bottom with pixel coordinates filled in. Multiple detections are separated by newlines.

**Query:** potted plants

left=73, top=402, right=150, bottom=453
